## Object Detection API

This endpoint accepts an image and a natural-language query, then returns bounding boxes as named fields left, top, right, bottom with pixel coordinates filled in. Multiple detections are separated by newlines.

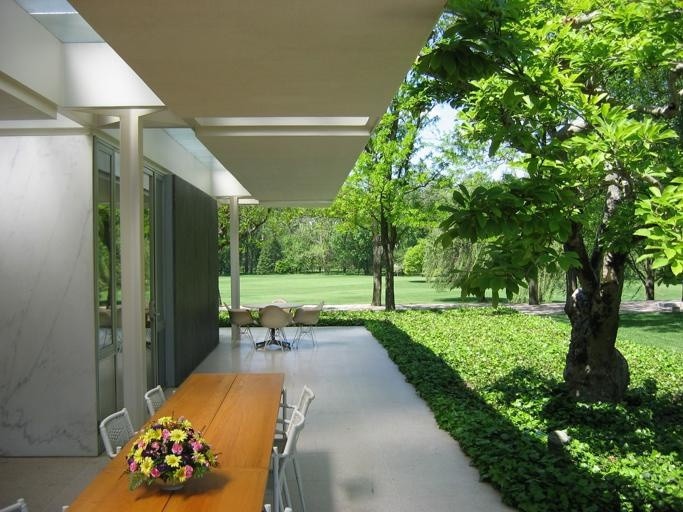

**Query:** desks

left=62, top=372, right=286, bottom=511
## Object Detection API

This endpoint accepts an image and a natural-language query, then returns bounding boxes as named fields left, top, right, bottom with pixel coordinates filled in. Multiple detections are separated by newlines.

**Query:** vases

left=158, top=484, right=185, bottom=492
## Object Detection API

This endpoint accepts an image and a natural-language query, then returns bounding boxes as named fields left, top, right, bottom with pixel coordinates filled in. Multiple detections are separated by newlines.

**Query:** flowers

left=126, top=413, right=221, bottom=490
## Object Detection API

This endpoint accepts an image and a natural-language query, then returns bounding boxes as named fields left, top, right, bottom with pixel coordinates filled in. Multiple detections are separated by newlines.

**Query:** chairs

left=99, top=384, right=167, bottom=458
left=261, top=385, right=317, bottom=510
left=223, top=299, right=326, bottom=353
